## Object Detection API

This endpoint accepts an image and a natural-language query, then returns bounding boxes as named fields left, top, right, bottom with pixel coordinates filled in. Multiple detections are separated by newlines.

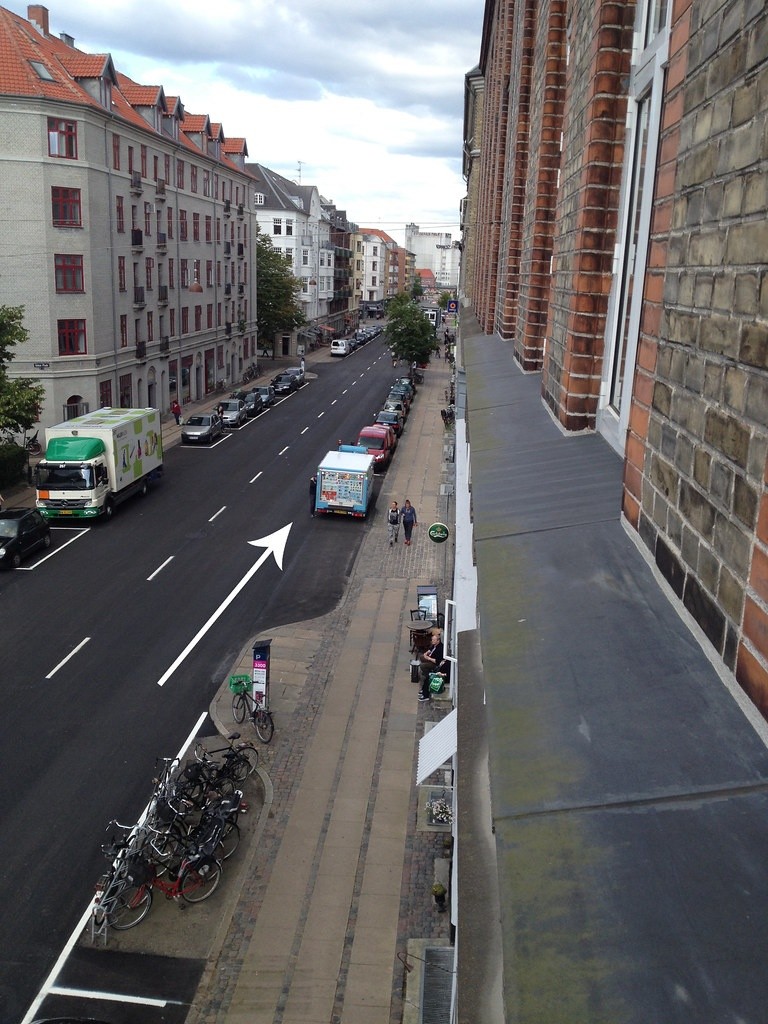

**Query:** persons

left=419, top=634, right=443, bottom=677
left=172, top=400, right=181, bottom=427
left=387, top=501, right=400, bottom=548
left=435, top=345, right=441, bottom=358
left=418, top=648, right=450, bottom=701
left=446, top=327, right=449, bottom=333
left=391, top=351, right=398, bottom=366
left=400, top=500, right=417, bottom=546
left=261, top=346, right=270, bottom=357
left=444, top=333, right=450, bottom=341
left=309, top=475, right=317, bottom=518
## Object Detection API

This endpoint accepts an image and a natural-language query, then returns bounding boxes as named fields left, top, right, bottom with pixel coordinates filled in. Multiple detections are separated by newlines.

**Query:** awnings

left=300, top=329, right=320, bottom=338
left=320, top=325, right=336, bottom=331
left=416, top=707, right=458, bottom=786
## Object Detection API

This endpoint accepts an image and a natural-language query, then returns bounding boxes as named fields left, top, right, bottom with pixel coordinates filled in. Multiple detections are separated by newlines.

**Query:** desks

left=405, top=620, right=434, bottom=630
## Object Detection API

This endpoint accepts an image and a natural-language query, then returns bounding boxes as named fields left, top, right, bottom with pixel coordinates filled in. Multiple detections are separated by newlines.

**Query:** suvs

left=0, top=507, right=52, bottom=571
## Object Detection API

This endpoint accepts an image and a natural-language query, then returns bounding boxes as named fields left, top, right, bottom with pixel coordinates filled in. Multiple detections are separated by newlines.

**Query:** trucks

left=29, top=407, right=163, bottom=523
left=315, top=439, right=376, bottom=521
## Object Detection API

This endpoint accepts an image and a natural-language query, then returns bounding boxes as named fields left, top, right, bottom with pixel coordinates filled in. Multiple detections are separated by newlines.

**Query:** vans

left=331, top=339, right=349, bottom=357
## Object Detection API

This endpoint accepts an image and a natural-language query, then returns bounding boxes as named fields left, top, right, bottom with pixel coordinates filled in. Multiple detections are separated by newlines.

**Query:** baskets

left=229, top=674, right=253, bottom=693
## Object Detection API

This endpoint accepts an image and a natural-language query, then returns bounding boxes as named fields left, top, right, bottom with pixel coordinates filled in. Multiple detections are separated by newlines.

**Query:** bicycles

left=220, top=416, right=231, bottom=434
left=242, top=363, right=264, bottom=385
left=217, top=378, right=233, bottom=395
left=409, top=368, right=425, bottom=383
left=0, top=429, right=41, bottom=456
left=399, top=357, right=406, bottom=367
left=94, top=732, right=258, bottom=930
left=229, top=673, right=274, bottom=744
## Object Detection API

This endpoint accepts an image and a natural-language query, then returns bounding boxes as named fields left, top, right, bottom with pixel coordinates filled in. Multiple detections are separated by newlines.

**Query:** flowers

left=424, top=799, right=454, bottom=825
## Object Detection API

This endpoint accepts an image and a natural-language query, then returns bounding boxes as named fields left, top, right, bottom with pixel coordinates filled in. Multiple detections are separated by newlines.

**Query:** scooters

left=392, top=358, right=397, bottom=368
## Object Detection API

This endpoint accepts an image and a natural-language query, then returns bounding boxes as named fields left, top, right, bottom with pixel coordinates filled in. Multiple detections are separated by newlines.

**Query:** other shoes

left=395, top=538, right=397, bottom=542
left=404, top=540, right=410, bottom=546
left=390, top=541, right=393, bottom=545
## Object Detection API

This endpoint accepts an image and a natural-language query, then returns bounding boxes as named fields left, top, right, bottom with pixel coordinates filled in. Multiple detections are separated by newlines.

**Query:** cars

left=351, top=375, right=416, bottom=472
left=181, top=413, right=221, bottom=444
left=283, top=367, right=304, bottom=387
left=270, top=374, right=297, bottom=394
left=235, top=391, right=262, bottom=417
left=251, top=385, right=275, bottom=408
left=214, top=398, right=247, bottom=428
left=347, top=324, right=382, bottom=353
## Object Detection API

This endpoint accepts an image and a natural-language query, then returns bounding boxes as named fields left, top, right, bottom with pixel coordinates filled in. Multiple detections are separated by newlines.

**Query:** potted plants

left=431, top=884, right=448, bottom=913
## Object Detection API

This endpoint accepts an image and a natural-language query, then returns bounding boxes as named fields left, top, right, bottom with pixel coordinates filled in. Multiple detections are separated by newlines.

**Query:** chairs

left=410, top=631, right=432, bottom=661
left=436, top=613, right=445, bottom=630
left=410, top=609, right=426, bottom=646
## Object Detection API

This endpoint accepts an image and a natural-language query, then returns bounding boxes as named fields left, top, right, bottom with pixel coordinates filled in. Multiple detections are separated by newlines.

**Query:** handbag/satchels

left=430, top=676, right=443, bottom=691
left=179, top=415, right=184, bottom=425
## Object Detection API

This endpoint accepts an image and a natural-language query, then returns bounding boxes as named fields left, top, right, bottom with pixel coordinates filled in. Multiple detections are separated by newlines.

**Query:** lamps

left=396, top=952, right=455, bottom=974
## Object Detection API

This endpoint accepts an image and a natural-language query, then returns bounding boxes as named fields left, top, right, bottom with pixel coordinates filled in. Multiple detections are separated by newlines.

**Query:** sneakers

left=418, top=696, right=430, bottom=701
left=417, top=690, right=423, bottom=695
